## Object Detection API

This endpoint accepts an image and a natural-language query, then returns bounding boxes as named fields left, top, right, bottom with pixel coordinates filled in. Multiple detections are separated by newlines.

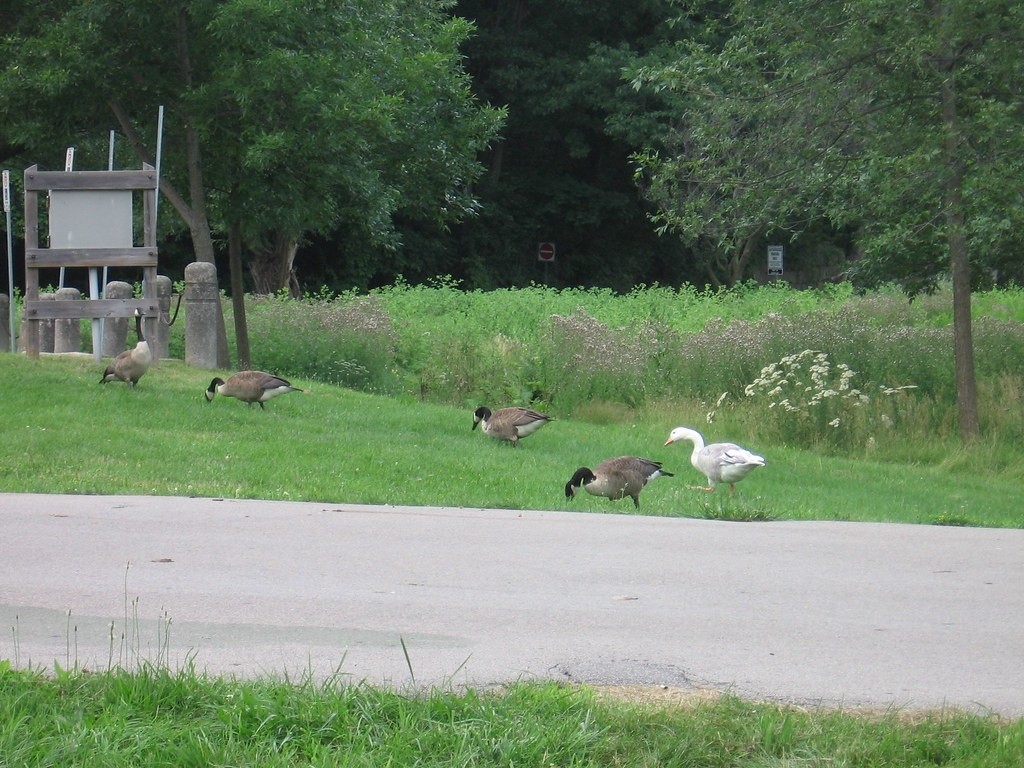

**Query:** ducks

left=664, top=427, right=765, bottom=497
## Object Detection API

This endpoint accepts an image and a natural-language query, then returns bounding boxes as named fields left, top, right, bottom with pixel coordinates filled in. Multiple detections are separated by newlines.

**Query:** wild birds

left=205, top=371, right=304, bottom=411
left=471, top=407, right=555, bottom=447
left=565, top=456, right=675, bottom=511
left=99, top=341, right=153, bottom=388
